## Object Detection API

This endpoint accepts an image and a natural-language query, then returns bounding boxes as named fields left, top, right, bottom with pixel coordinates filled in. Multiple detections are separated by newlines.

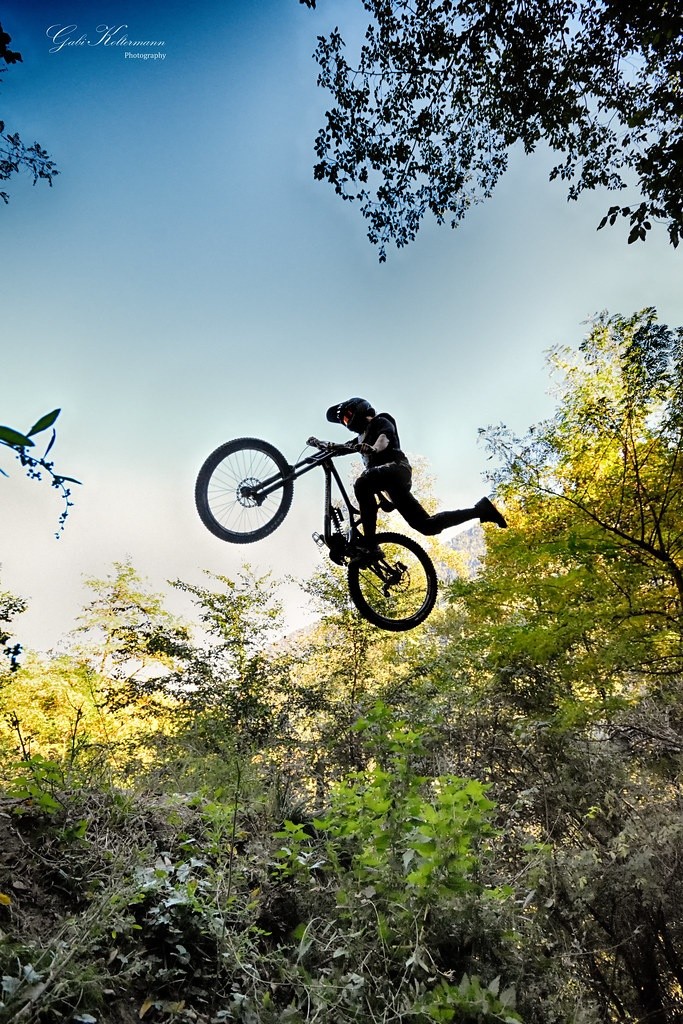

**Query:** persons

left=306, top=398, right=508, bottom=566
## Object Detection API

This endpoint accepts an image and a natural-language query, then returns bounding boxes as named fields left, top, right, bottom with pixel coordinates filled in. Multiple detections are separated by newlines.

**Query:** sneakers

left=475, top=496, right=508, bottom=529
left=349, top=547, right=386, bottom=570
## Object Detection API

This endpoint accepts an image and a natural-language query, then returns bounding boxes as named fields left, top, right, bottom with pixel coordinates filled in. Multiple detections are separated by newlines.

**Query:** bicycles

left=194, top=438, right=439, bottom=633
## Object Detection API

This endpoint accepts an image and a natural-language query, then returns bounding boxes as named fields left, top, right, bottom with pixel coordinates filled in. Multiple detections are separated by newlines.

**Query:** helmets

left=325, top=397, right=376, bottom=432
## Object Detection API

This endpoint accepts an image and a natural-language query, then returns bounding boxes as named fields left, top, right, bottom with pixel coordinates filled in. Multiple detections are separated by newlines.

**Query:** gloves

left=353, top=443, right=376, bottom=456
